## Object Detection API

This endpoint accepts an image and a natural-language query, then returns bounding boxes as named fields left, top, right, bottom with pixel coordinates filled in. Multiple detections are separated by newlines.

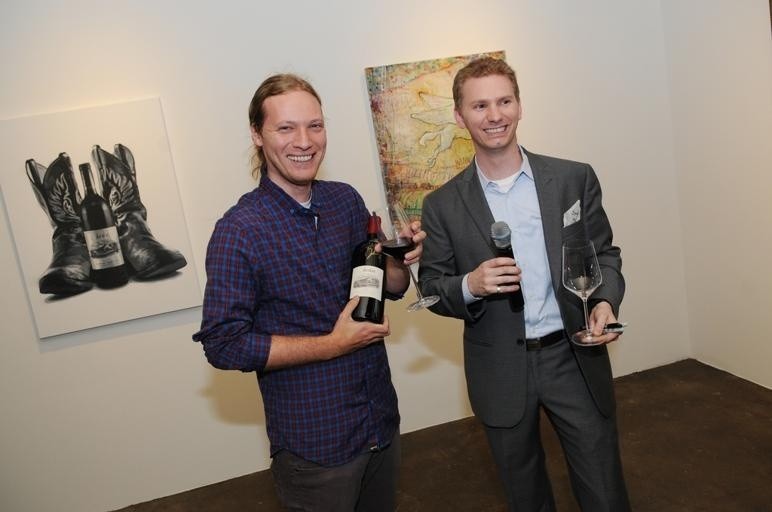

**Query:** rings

left=497, top=286, right=500, bottom=292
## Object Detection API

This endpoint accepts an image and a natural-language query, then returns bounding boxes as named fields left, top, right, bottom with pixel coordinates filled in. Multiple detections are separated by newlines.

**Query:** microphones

left=490, top=221, right=524, bottom=314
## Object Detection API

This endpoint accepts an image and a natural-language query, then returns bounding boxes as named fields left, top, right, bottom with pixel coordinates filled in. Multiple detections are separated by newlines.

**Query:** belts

left=526, top=329, right=566, bottom=349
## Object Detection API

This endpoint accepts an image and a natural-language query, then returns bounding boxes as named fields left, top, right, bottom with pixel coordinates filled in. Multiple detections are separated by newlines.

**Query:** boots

left=25, top=144, right=187, bottom=294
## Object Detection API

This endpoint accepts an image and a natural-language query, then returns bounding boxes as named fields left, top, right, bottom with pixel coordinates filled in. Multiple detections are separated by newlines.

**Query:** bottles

left=78, top=163, right=129, bottom=290
left=347, top=216, right=388, bottom=323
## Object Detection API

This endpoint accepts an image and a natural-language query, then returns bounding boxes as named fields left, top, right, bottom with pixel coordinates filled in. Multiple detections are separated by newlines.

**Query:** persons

left=420, top=58, right=631, bottom=512
left=192, top=74, right=426, bottom=511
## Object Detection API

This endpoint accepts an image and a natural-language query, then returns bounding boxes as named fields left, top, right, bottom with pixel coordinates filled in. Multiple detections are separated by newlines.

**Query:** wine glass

left=378, top=202, right=441, bottom=311
left=561, top=240, right=608, bottom=346
